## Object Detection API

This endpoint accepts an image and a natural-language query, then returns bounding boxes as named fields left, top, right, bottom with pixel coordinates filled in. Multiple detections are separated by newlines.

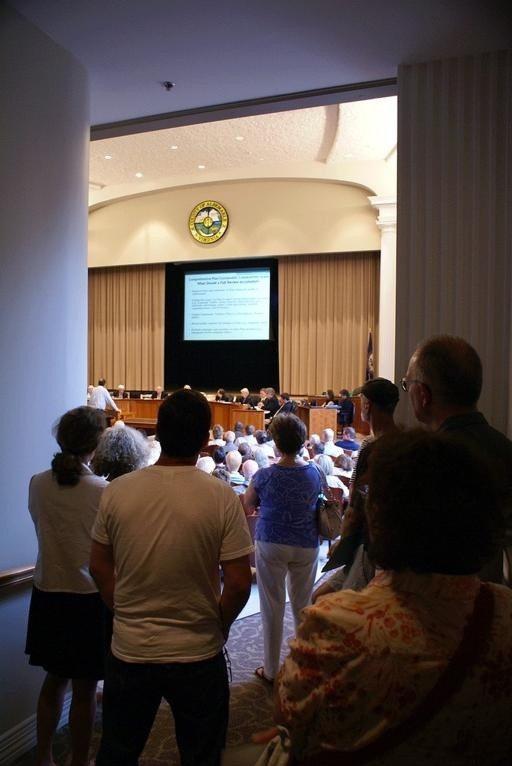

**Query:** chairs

left=321, top=486, right=344, bottom=551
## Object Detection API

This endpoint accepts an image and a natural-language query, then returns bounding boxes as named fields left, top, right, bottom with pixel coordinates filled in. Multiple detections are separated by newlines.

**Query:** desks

left=298, top=395, right=370, bottom=443
left=112, top=398, right=166, bottom=420
left=208, top=401, right=265, bottom=432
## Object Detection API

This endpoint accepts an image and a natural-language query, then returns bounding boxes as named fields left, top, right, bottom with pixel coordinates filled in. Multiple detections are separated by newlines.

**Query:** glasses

left=400, top=376, right=431, bottom=397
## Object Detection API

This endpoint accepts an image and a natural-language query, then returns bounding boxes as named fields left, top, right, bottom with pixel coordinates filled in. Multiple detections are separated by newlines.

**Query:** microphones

left=249, top=397, right=258, bottom=411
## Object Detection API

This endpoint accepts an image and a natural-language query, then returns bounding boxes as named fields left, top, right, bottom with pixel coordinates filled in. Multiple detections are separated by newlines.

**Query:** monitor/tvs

left=107, top=389, right=119, bottom=398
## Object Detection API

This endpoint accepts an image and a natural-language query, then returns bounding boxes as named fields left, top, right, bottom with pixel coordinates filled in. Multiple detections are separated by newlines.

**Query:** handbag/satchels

left=257, top=726, right=293, bottom=766
left=318, top=497, right=344, bottom=540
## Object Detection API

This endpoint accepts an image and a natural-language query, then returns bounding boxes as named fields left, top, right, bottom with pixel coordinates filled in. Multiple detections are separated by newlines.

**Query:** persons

left=21, top=406, right=112, bottom=765
left=86, top=378, right=366, bottom=500
left=274, top=426, right=512, bottom=765
left=85, top=389, right=254, bottom=765
left=401, top=333, right=509, bottom=585
left=310, top=376, right=400, bottom=606
left=241, top=413, right=327, bottom=685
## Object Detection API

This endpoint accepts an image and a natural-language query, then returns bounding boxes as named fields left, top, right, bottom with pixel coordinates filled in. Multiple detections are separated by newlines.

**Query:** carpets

left=14, top=567, right=343, bottom=764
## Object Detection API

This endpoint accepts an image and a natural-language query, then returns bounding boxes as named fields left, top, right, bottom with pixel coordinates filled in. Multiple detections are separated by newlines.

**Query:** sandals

left=256, top=665, right=274, bottom=684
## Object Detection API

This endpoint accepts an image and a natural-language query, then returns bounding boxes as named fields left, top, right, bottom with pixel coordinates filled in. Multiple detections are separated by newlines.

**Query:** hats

left=352, top=377, right=400, bottom=405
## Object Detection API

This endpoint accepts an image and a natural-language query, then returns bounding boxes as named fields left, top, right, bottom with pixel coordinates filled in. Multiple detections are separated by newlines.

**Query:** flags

left=366, top=333, right=375, bottom=382
